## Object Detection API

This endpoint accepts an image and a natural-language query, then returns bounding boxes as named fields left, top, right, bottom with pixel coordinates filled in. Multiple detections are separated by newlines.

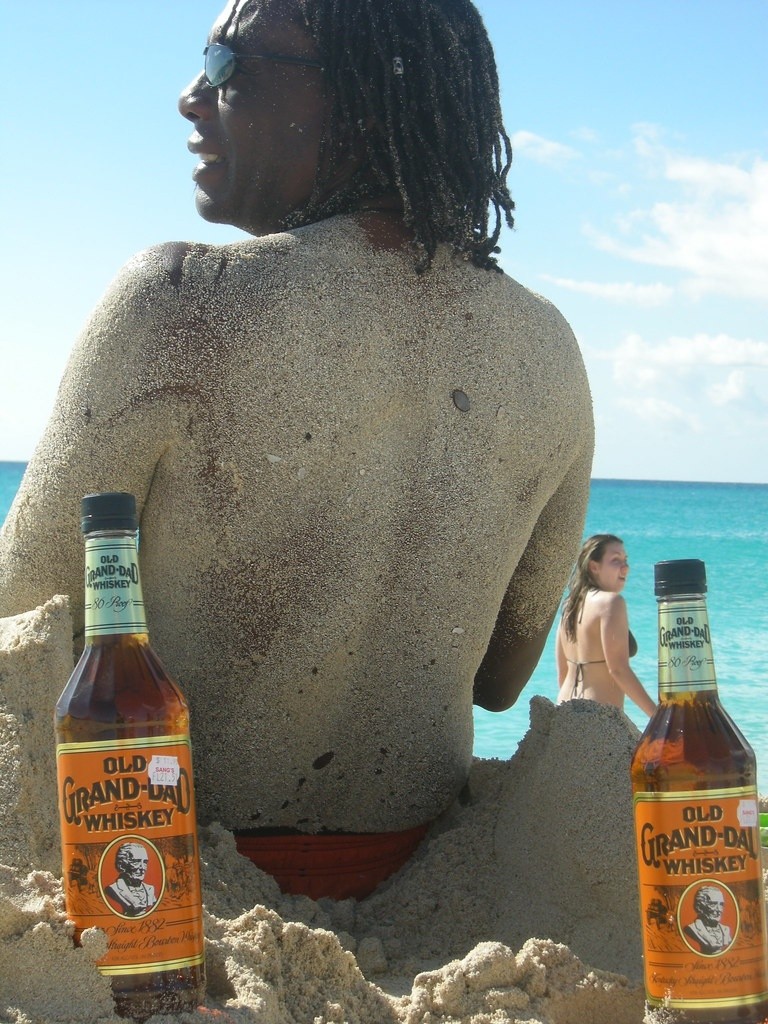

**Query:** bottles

left=52, top=491, right=207, bottom=1020
left=630, top=559, right=768, bottom=1024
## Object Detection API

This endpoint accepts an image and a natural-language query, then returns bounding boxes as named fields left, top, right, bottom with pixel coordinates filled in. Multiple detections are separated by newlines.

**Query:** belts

left=237, top=824, right=429, bottom=904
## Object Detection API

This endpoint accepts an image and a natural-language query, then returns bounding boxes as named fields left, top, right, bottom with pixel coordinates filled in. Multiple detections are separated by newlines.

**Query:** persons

left=554, top=535, right=658, bottom=723
left=0, top=0, right=595, bottom=903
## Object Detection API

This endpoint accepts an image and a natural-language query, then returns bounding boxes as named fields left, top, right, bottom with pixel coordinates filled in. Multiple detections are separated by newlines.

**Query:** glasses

left=203, top=42, right=356, bottom=88
